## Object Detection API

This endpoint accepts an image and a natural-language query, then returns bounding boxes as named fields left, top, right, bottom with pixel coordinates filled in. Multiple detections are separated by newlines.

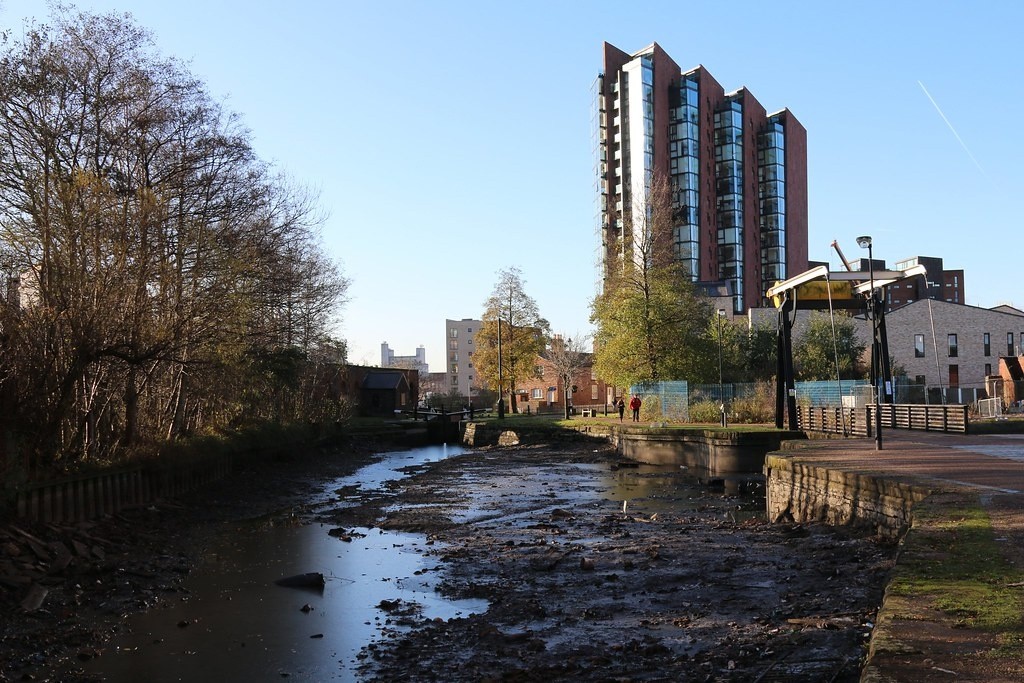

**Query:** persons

left=630, top=394, right=641, bottom=422
left=612, top=397, right=617, bottom=410
left=618, top=401, right=625, bottom=422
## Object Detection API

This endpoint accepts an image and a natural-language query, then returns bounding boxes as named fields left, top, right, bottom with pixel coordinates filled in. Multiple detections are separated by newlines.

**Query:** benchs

left=582, top=408, right=597, bottom=417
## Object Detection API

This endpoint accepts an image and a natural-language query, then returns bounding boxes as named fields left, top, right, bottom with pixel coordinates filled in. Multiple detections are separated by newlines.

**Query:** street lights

left=497, top=308, right=507, bottom=418
left=717, top=308, right=726, bottom=428
left=856, top=236, right=882, bottom=451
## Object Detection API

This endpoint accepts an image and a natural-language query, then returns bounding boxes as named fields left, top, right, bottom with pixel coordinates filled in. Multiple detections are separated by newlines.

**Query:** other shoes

left=637, top=420, right=639, bottom=423
left=633, top=421, right=635, bottom=423
